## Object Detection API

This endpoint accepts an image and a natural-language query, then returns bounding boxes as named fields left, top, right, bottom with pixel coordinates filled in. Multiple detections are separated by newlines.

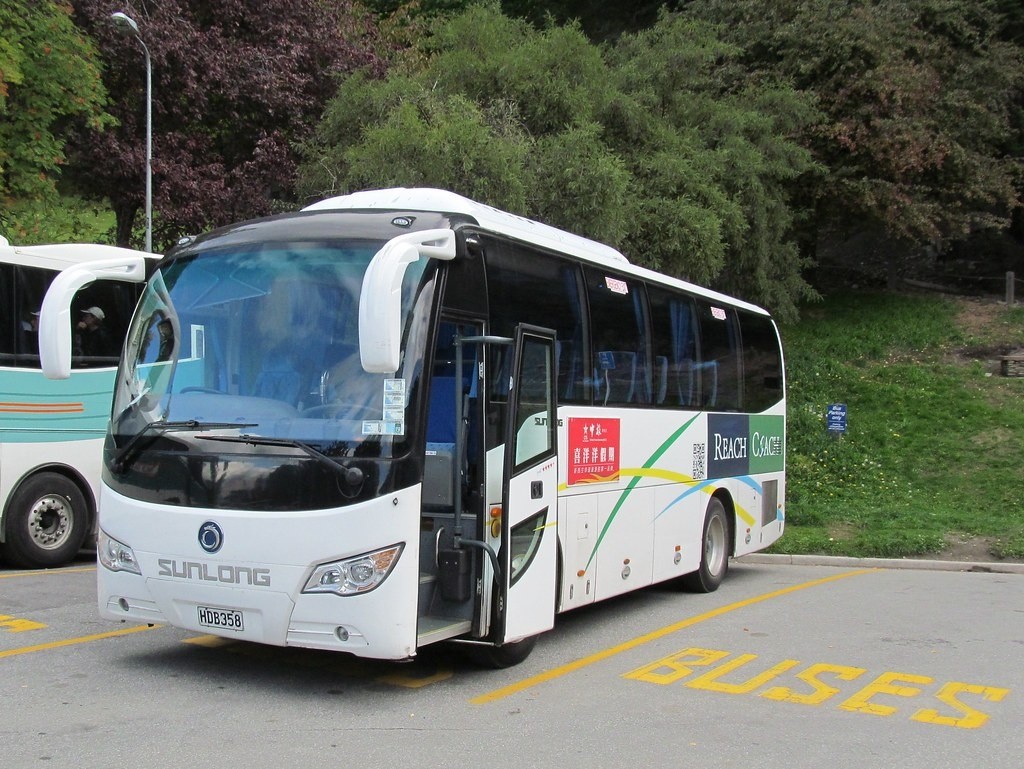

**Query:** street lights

left=109, top=10, right=154, bottom=254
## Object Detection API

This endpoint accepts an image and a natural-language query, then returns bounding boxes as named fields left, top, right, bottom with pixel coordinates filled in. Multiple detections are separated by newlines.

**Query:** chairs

left=421, top=367, right=476, bottom=505
left=554, top=340, right=718, bottom=408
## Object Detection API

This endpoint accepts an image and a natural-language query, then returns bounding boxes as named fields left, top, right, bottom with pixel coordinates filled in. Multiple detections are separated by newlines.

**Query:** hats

left=80, top=306, right=105, bottom=322
left=30, top=310, right=41, bottom=317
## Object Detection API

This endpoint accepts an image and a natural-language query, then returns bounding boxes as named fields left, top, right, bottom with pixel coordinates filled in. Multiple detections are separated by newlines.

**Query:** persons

left=77, top=305, right=119, bottom=367
left=29, top=310, right=40, bottom=354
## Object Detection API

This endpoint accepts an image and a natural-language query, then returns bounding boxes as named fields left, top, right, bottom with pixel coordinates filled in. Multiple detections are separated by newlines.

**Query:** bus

left=34, top=186, right=788, bottom=673
left=0, top=235, right=251, bottom=571
